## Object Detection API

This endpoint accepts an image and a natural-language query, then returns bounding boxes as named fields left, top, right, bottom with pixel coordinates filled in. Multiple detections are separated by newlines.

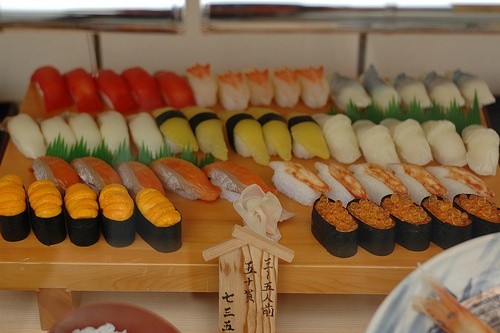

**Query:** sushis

left=30, top=63, right=495, bottom=113
left=0, top=175, right=182, bottom=253
left=28, top=156, right=278, bottom=202
left=268, top=160, right=495, bottom=206
left=0, top=105, right=500, bottom=176
left=311, top=194, right=500, bottom=258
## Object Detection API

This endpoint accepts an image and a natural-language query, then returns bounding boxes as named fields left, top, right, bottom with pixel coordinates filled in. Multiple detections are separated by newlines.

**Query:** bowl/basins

left=46, top=300, right=184, bottom=333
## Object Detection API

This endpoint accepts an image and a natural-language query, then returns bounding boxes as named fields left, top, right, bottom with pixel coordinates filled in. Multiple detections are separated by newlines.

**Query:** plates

left=364, top=228, right=500, bottom=333
